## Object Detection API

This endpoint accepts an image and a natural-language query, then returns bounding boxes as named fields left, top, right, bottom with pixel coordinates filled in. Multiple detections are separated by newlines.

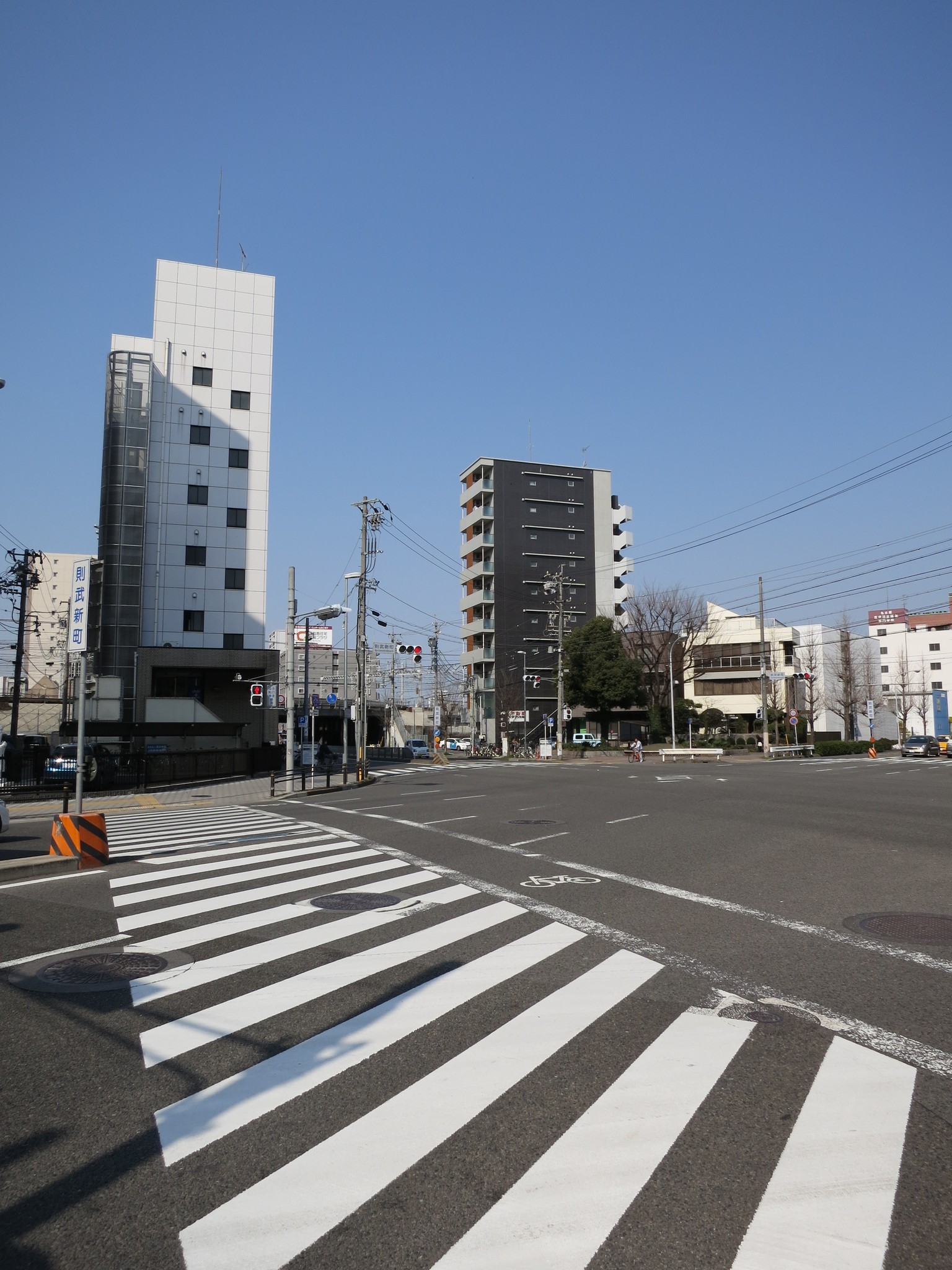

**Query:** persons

left=318, top=738, right=337, bottom=771
left=629, top=738, right=643, bottom=763
left=690, top=738, right=702, bottom=757
left=512, top=737, right=519, bottom=745
left=756, top=734, right=763, bottom=753
left=527, top=736, right=540, bottom=751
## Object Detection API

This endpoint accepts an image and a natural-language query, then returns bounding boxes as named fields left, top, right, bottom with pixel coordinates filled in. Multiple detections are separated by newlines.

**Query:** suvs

left=6, top=735, right=51, bottom=774
left=573, top=733, right=602, bottom=747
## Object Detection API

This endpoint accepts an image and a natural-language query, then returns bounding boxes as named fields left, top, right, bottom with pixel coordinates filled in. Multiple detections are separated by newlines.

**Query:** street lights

left=894, top=670, right=921, bottom=747
left=517, top=651, right=526, bottom=758
left=670, top=633, right=688, bottom=749
left=342, top=572, right=361, bottom=784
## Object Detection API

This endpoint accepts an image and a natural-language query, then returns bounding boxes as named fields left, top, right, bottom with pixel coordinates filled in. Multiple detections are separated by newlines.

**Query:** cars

left=936, top=735, right=951, bottom=754
left=43, top=743, right=118, bottom=789
left=438, top=738, right=471, bottom=751
left=405, top=739, right=429, bottom=758
left=462, top=738, right=472, bottom=743
left=475, top=738, right=484, bottom=745
left=945, top=736, right=952, bottom=758
left=901, top=735, right=939, bottom=757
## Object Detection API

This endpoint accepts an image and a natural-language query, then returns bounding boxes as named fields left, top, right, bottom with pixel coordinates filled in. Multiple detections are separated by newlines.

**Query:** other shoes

left=640, top=759, right=643, bottom=762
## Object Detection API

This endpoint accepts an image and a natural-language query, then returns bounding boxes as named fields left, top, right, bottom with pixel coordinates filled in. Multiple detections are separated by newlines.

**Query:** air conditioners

left=161, top=640, right=179, bottom=647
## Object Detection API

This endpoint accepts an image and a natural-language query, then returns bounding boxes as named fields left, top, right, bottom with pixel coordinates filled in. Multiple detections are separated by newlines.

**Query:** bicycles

left=466, top=745, right=503, bottom=757
left=628, top=747, right=644, bottom=763
left=513, top=743, right=539, bottom=759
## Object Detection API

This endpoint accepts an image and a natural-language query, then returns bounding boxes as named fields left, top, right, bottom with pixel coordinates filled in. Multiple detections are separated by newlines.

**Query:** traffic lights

left=524, top=675, right=540, bottom=688
left=567, top=709, right=572, bottom=719
left=341, top=606, right=351, bottom=615
left=395, top=645, right=422, bottom=663
left=793, top=673, right=812, bottom=679
left=250, top=684, right=263, bottom=706
left=756, top=708, right=762, bottom=719
